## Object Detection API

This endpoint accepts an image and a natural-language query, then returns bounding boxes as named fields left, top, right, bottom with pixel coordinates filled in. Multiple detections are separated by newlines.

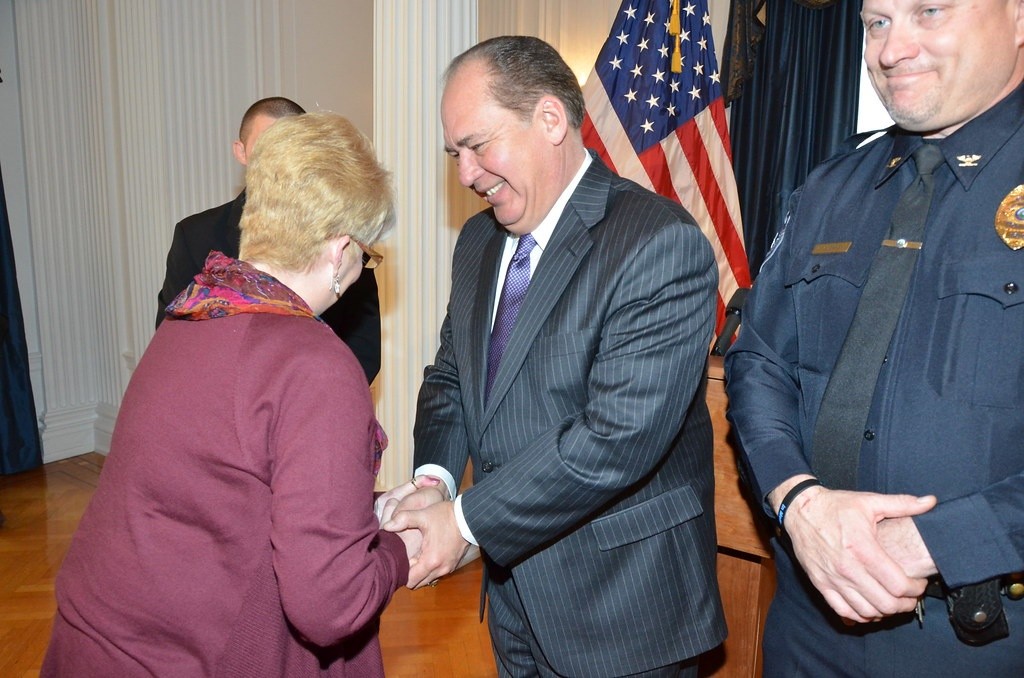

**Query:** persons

left=723, top=0, right=1024, bottom=678
left=156, top=97, right=381, bottom=387
left=383, top=36, right=728, bottom=678
left=41, top=111, right=440, bottom=678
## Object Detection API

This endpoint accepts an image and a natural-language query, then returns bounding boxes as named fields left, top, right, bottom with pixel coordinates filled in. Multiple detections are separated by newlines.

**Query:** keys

left=914, top=598, right=926, bottom=622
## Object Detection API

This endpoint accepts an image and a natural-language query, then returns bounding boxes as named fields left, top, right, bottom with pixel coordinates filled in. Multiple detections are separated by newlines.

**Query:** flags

left=580, top=0, right=751, bottom=353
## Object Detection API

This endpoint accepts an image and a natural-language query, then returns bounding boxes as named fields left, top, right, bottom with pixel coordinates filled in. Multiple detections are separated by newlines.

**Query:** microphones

left=710, top=288, right=750, bottom=357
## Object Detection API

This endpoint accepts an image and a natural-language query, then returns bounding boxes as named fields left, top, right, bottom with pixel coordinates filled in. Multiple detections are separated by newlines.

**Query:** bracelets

left=778, top=479, right=822, bottom=526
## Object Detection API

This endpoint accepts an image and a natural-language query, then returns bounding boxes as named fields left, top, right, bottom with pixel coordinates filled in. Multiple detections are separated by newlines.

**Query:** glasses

left=334, top=232, right=384, bottom=269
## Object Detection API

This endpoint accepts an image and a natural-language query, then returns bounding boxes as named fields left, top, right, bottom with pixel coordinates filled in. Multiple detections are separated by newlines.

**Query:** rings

left=429, top=579, right=440, bottom=588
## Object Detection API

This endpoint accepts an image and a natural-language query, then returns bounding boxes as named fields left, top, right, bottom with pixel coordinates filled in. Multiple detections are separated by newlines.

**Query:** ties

left=809, top=143, right=945, bottom=492
left=482, top=232, right=537, bottom=413
